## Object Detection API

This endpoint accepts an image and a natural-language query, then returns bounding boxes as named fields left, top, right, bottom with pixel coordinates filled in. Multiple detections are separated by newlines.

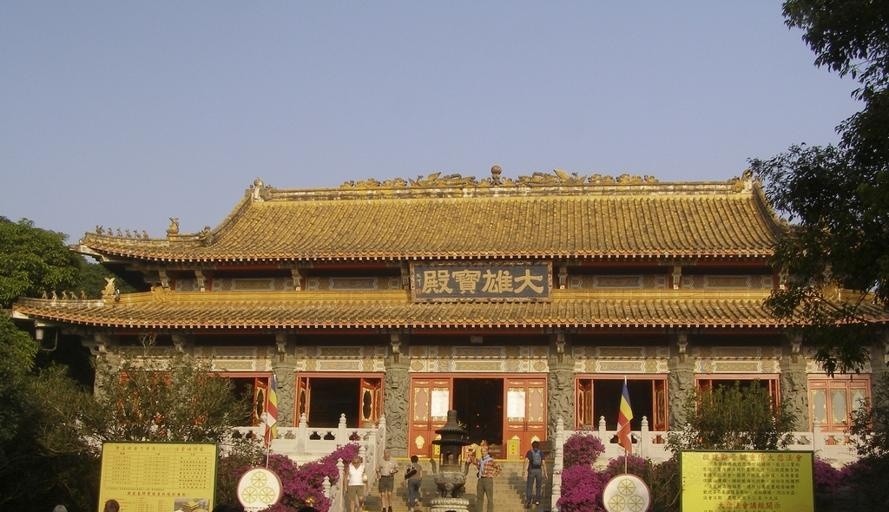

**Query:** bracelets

left=523, top=471, right=527, bottom=474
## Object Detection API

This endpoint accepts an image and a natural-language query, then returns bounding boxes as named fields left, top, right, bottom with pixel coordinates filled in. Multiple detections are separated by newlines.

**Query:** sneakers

left=525, top=500, right=540, bottom=508
left=382, top=508, right=393, bottom=512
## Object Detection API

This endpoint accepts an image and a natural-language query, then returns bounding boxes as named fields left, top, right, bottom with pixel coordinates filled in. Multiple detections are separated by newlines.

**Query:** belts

left=381, top=475, right=394, bottom=477
left=478, top=476, right=493, bottom=479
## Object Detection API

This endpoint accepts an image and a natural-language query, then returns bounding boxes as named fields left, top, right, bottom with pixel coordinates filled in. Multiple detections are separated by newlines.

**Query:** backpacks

left=531, top=449, right=542, bottom=469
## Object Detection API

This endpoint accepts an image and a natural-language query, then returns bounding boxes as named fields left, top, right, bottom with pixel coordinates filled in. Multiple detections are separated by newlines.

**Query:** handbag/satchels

left=404, top=462, right=417, bottom=480
left=363, top=473, right=367, bottom=482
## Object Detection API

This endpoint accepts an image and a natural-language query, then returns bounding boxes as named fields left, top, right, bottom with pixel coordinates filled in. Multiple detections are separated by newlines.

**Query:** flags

left=264, top=373, right=279, bottom=449
left=616, top=384, right=634, bottom=457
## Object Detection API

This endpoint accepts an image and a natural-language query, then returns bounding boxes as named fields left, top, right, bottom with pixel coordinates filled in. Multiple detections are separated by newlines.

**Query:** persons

left=522, top=435, right=548, bottom=508
left=466, top=445, right=502, bottom=512
left=344, top=456, right=369, bottom=512
left=375, top=448, right=399, bottom=512
left=103, top=499, right=119, bottom=512
left=463, top=444, right=480, bottom=476
left=54, top=505, right=67, bottom=512
left=404, top=455, right=423, bottom=512
